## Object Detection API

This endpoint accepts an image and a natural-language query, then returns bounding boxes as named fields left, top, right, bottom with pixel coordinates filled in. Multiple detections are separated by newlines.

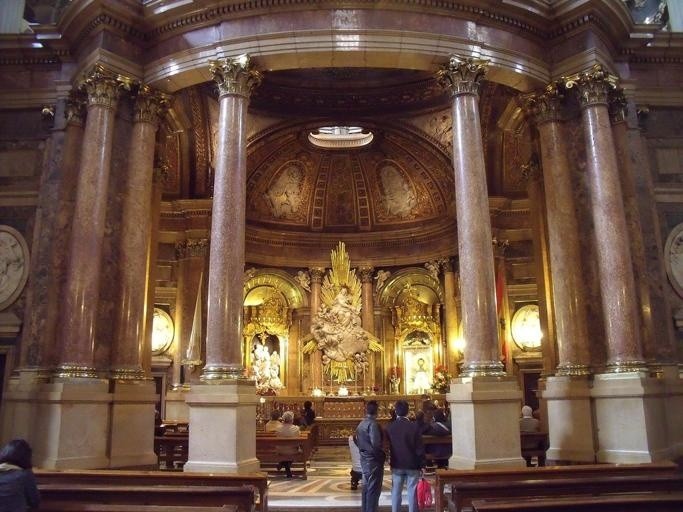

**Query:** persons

left=0, top=439, right=44, bottom=511
left=303, top=400, right=315, bottom=419
left=353, top=400, right=386, bottom=511
left=295, top=271, right=310, bottom=292
left=416, top=394, right=451, bottom=470
left=424, top=262, right=439, bottom=284
left=311, top=289, right=369, bottom=381
left=250, top=344, right=281, bottom=388
left=275, top=412, right=300, bottom=477
left=519, top=406, right=539, bottom=433
left=347, top=420, right=363, bottom=490
left=294, top=409, right=312, bottom=427
left=264, top=409, right=282, bottom=433
left=382, top=401, right=425, bottom=511
left=373, top=270, right=390, bottom=293
left=243, top=267, right=259, bottom=283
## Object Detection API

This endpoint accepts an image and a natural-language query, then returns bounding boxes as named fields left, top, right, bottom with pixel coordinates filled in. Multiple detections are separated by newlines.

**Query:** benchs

left=417, top=468, right=682, bottom=512
left=33, top=468, right=271, bottom=512
left=383, top=430, right=550, bottom=469
left=153, top=426, right=318, bottom=479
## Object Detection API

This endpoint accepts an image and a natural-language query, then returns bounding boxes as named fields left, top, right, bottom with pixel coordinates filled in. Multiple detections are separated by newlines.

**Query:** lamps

left=311, top=387, right=348, bottom=397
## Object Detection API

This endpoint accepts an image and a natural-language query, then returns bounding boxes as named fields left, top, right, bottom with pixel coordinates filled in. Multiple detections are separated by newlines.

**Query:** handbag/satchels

left=416, top=479, right=433, bottom=510
